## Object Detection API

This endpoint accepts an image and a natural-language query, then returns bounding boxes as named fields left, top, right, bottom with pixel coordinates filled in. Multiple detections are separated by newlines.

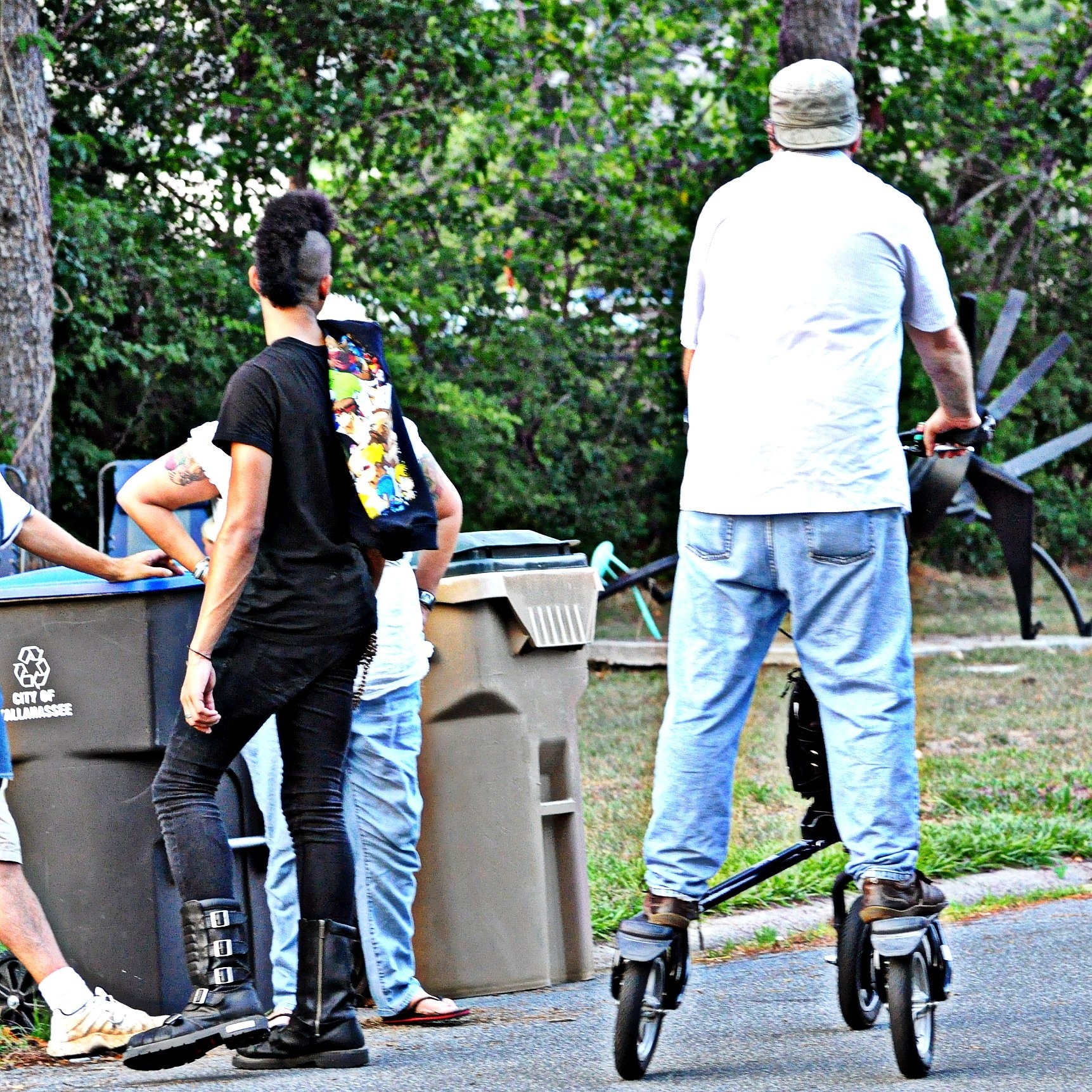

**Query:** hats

left=769, top=59, right=859, bottom=149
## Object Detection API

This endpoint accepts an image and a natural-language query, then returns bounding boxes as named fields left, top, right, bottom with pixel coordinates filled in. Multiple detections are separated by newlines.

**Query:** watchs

left=418, top=587, right=436, bottom=609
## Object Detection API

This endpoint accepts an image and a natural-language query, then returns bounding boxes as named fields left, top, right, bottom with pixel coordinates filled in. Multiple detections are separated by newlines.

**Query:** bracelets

left=186, top=645, right=212, bottom=662
left=192, top=557, right=210, bottom=579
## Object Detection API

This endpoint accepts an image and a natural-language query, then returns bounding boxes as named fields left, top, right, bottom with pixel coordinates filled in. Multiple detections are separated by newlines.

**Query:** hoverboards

left=610, top=409, right=1092, bottom=1080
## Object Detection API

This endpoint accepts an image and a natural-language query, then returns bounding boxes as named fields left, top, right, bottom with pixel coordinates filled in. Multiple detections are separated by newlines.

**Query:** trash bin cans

left=409, top=524, right=607, bottom=997
left=0, top=562, right=275, bottom=1018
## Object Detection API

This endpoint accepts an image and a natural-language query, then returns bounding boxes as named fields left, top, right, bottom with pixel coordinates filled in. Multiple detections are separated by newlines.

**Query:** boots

left=234, top=918, right=369, bottom=1070
left=122, top=898, right=269, bottom=1071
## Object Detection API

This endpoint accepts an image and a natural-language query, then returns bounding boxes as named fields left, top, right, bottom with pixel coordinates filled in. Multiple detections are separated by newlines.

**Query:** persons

left=118, top=190, right=381, bottom=1072
left=0, top=465, right=184, bottom=1060
left=635, top=57, right=983, bottom=925
left=115, top=289, right=471, bottom=1025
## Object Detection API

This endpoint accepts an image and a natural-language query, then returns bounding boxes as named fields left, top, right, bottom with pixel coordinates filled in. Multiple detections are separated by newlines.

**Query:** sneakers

left=860, top=869, right=949, bottom=924
left=644, top=888, right=698, bottom=927
left=46, top=986, right=170, bottom=1060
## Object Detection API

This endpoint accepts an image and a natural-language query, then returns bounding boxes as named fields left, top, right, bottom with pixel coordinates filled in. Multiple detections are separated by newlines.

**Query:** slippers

left=382, top=994, right=470, bottom=1024
